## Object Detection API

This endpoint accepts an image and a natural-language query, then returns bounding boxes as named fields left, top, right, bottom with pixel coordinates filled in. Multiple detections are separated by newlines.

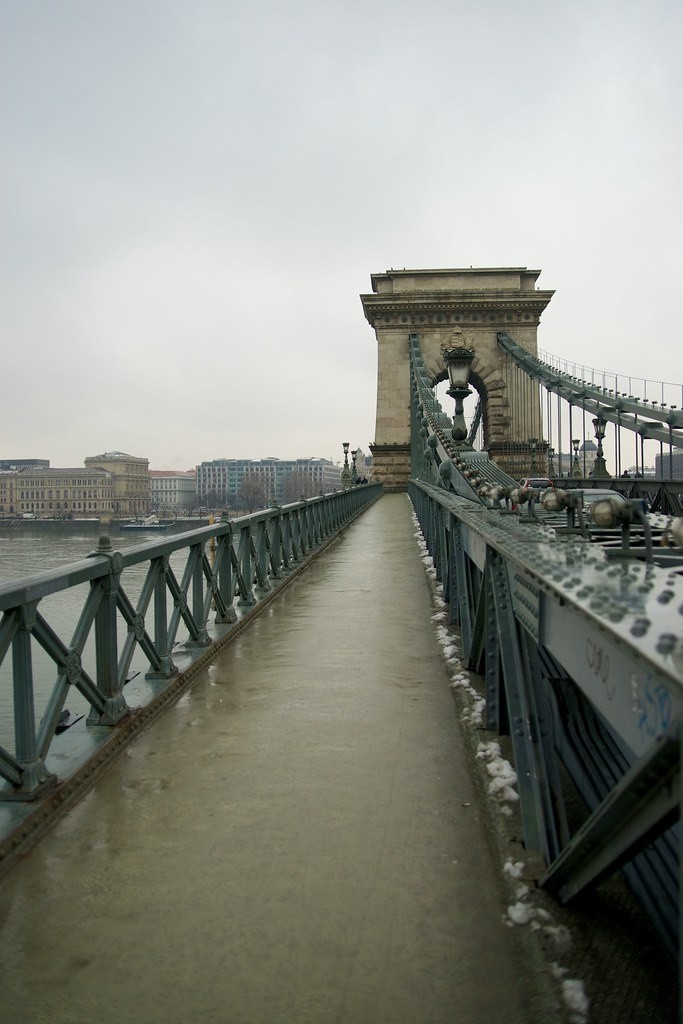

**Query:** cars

left=565, top=489, right=628, bottom=509
left=519, top=478, right=555, bottom=492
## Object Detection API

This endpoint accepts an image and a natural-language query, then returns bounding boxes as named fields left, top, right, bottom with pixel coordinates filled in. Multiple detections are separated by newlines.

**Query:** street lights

left=351, top=451, right=358, bottom=484
left=341, top=442, right=352, bottom=486
left=592, top=419, right=609, bottom=476
left=443, top=347, right=476, bottom=442
left=529, top=438, right=539, bottom=476
left=570, top=439, right=581, bottom=477
left=548, top=448, right=555, bottom=478
